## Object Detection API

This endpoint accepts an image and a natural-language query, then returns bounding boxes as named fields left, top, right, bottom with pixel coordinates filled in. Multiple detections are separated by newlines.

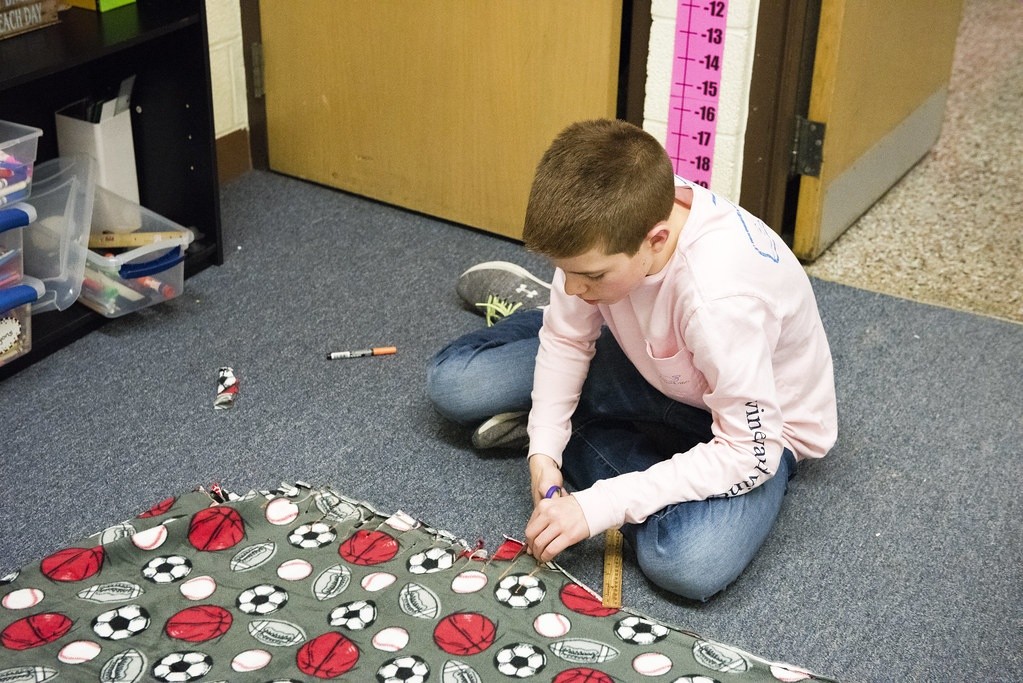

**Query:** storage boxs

left=0, top=121, right=194, bottom=365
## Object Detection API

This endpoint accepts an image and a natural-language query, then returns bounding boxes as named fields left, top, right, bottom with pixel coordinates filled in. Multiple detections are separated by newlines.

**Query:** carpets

left=0, top=170, right=1023, bottom=683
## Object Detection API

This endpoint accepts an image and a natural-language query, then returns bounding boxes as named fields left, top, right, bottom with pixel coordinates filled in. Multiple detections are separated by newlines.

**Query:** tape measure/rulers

left=663, top=1, right=730, bottom=191
left=88, top=231, right=188, bottom=248
left=601, top=522, right=625, bottom=609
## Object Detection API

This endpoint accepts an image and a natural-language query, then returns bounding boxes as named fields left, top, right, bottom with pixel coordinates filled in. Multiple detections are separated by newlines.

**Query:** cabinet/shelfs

left=0, top=0, right=223, bottom=382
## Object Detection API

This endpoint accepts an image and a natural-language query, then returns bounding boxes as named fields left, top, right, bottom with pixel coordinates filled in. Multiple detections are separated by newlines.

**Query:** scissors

left=536, top=485, right=563, bottom=569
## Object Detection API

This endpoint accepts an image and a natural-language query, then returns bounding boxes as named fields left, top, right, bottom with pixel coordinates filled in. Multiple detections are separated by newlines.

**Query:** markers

left=53, top=254, right=174, bottom=316
left=0, top=149, right=32, bottom=206
left=326, top=346, right=398, bottom=361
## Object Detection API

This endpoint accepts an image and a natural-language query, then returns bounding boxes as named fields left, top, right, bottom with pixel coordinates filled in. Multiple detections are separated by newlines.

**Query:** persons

left=421, top=116, right=843, bottom=605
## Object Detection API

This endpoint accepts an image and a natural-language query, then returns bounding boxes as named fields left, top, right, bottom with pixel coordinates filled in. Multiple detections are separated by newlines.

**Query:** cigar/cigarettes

left=323, top=344, right=398, bottom=363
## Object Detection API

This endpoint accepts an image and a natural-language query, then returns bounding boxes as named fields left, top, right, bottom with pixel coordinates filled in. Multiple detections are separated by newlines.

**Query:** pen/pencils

left=0, top=241, right=22, bottom=290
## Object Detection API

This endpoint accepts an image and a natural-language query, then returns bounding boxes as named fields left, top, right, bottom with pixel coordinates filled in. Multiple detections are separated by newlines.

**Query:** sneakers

left=456, top=261, right=551, bottom=323
left=471, top=411, right=531, bottom=451
